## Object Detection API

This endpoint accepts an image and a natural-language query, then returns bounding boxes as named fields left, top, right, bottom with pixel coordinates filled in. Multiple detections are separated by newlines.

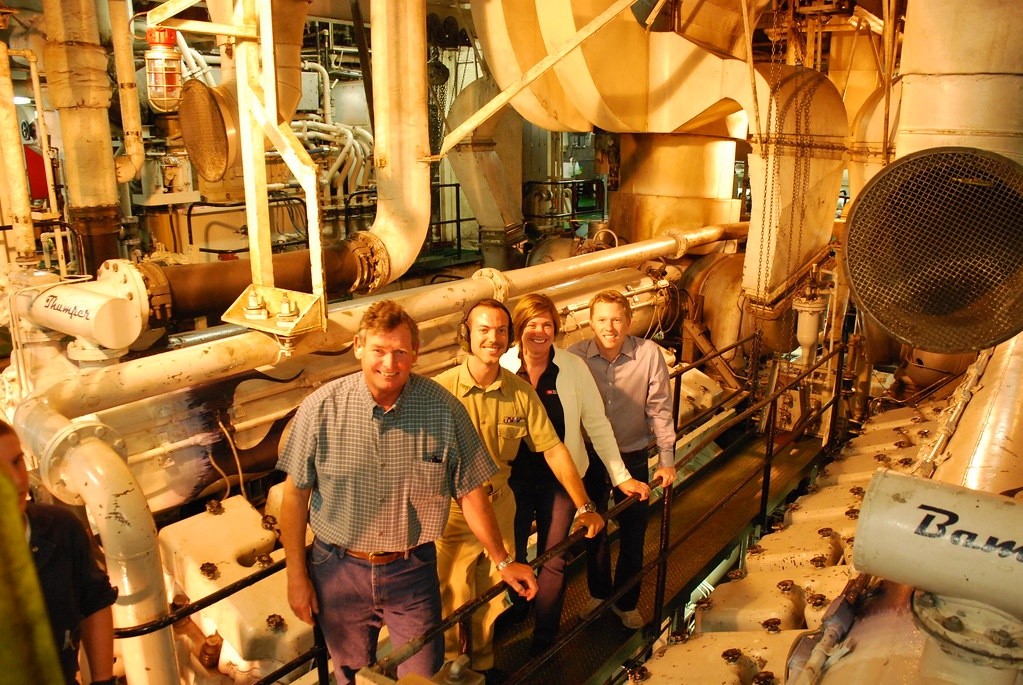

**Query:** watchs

left=92, top=675, right=119, bottom=685
left=496, top=553, right=515, bottom=572
left=578, top=500, right=597, bottom=515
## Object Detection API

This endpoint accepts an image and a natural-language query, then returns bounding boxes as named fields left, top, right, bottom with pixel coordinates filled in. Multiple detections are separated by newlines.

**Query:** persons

left=566, top=290, right=676, bottom=630
left=0, top=419, right=124, bottom=685
left=276, top=299, right=538, bottom=685
left=430, top=298, right=605, bottom=685
left=494, top=294, right=652, bottom=656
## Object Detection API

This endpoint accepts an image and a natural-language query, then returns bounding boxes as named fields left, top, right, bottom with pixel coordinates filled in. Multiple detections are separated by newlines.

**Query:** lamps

left=143, top=27, right=184, bottom=114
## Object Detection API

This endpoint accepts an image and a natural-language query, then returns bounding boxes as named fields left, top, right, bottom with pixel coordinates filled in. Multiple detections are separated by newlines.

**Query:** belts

left=450, top=483, right=509, bottom=504
left=620, top=446, right=650, bottom=467
left=329, top=545, right=422, bottom=566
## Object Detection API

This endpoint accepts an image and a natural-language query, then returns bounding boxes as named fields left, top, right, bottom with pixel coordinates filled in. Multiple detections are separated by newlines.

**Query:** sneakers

left=579, top=597, right=605, bottom=619
left=611, top=602, right=644, bottom=628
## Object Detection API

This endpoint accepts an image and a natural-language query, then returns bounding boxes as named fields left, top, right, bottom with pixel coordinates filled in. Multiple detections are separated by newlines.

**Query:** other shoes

left=509, top=605, right=532, bottom=626
left=527, top=635, right=554, bottom=660
left=483, top=667, right=509, bottom=685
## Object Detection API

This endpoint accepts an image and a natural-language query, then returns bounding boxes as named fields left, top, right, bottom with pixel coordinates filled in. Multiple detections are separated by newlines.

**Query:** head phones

left=457, top=298, right=514, bottom=353
